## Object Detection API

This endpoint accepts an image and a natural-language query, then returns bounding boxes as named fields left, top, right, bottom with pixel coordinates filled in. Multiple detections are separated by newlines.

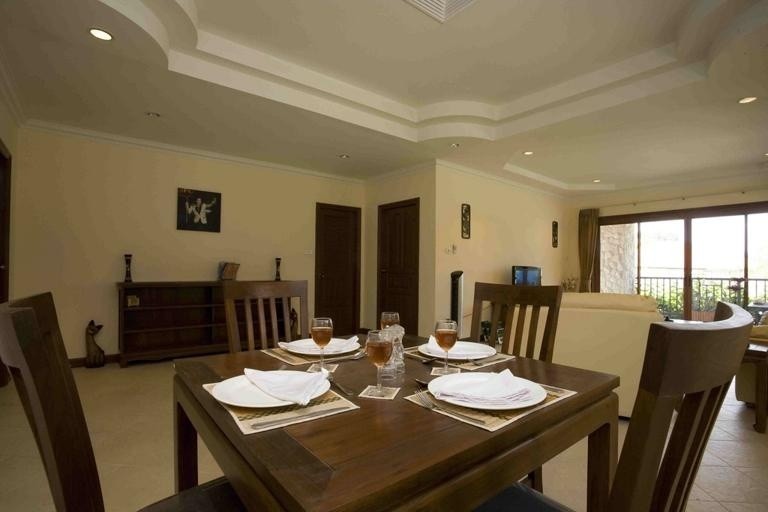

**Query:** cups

left=379, top=312, right=406, bottom=378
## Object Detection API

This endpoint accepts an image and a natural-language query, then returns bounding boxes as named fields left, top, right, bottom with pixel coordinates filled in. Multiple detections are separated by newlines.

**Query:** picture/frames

left=176, top=186, right=221, bottom=233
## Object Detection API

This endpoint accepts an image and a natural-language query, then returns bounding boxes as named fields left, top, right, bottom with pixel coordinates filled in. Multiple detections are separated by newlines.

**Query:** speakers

left=451, top=271, right=464, bottom=338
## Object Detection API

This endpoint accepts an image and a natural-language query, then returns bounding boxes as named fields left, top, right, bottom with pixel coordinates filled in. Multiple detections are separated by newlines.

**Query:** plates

left=211, top=370, right=332, bottom=407
left=287, top=336, right=361, bottom=358
left=428, top=371, right=547, bottom=410
left=416, top=339, right=496, bottom=362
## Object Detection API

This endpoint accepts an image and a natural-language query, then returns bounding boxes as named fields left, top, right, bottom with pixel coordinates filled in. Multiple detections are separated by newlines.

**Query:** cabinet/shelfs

left=115, top=281, right=291, bottom=368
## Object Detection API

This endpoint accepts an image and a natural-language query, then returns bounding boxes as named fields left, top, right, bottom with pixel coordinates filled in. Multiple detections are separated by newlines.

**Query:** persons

left=184, top=197, right=219, bottom=227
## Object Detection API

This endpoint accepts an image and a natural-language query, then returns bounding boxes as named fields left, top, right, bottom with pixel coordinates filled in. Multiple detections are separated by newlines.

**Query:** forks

left=415, top=387, right=488, bottom=425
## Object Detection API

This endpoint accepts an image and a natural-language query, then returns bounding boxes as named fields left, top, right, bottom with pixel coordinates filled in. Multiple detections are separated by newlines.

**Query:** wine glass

left=311, top=317, right=333, bottom=371
left=367, top=329, right=393, bottom=398
left=434, top=319, right=457, bottom=374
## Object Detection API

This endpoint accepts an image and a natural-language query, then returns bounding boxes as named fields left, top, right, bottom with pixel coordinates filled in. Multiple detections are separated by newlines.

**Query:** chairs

left=734, top=311, right=768, bottom=409
left=222, top=278, right=310, bottom=354
left=0, top=290, right=248, bottom=512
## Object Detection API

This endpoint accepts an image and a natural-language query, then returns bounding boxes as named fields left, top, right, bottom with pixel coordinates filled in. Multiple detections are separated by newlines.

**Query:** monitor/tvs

left=512, top=266, right=542, bottom=286
